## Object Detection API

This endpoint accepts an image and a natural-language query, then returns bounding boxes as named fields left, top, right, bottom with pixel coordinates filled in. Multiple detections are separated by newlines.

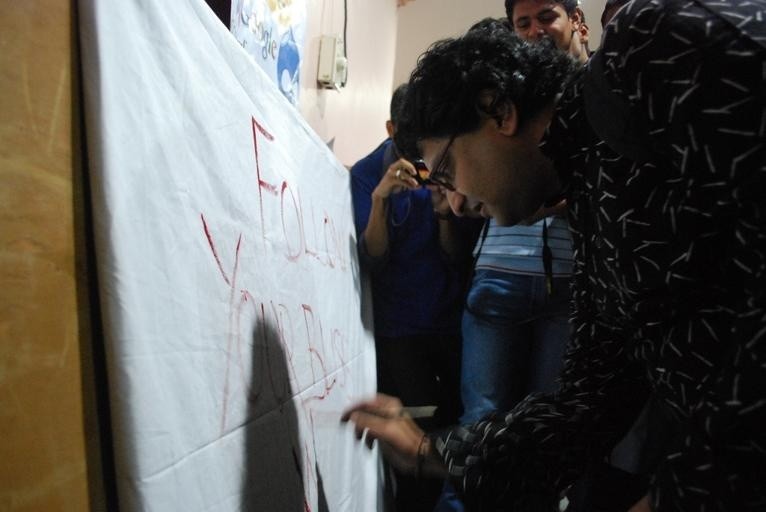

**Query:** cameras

left=404, top=158, right=440, bottom=188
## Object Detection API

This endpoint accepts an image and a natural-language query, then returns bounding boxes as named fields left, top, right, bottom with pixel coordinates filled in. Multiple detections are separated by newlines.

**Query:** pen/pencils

left=356, top=406, right=438, bottom=419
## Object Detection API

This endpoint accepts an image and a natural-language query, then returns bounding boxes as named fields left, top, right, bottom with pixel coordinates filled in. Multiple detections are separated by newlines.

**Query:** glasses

left=429, top=136, right=457, bottom=192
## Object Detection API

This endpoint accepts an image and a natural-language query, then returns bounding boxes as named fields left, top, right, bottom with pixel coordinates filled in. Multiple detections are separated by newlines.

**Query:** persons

left=337, top=0, right=766, bottom=512
